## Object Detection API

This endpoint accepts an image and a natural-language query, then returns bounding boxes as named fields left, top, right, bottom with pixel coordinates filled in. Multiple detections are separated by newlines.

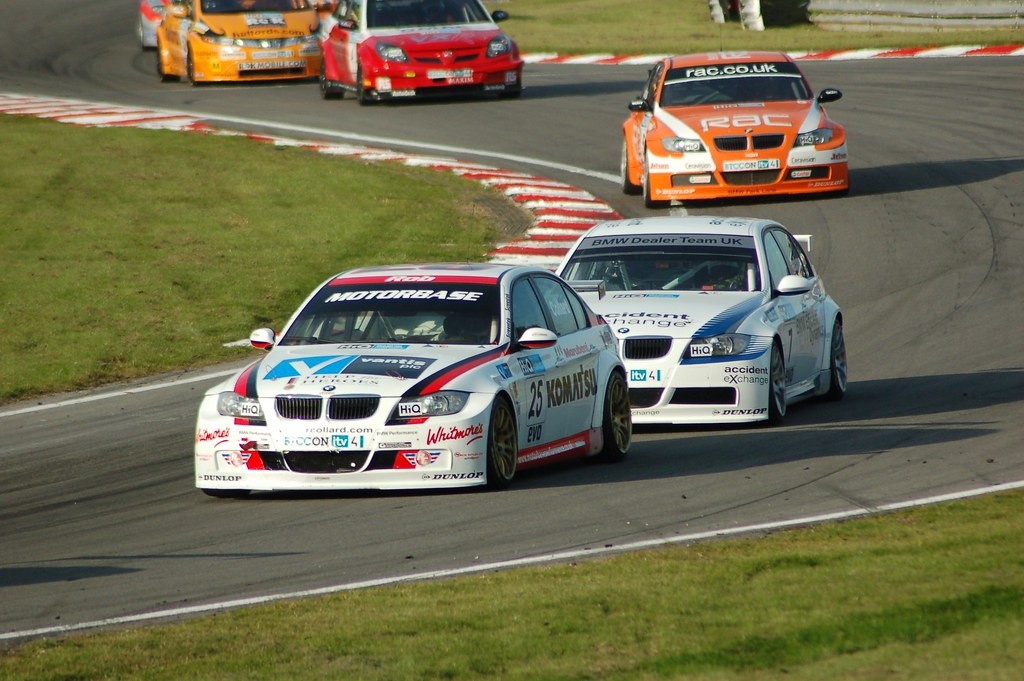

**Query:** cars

left=135, top=0, right=171, bottom=54
left=314, top=0, right=525, bottom=107
left=154, top=0, right=340, bottom=88
left=620, top=50, right=850, bottom=210
left=548, top=214, right=850, bottom=430
left=195, top=261, right=635, bottom=498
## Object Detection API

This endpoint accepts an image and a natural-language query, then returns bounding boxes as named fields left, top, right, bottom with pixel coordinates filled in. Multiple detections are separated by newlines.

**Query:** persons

left=708, top=260, right=741, bottom=289
left=422, top=0, right=452, bottom=22
left=442, top=302, right=492, bottom=343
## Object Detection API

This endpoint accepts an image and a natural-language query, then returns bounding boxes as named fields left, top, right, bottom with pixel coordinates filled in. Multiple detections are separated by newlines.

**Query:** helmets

left=707, top=259, right=739, bottom=288
left=453, top=296, right=496, bottom=338
